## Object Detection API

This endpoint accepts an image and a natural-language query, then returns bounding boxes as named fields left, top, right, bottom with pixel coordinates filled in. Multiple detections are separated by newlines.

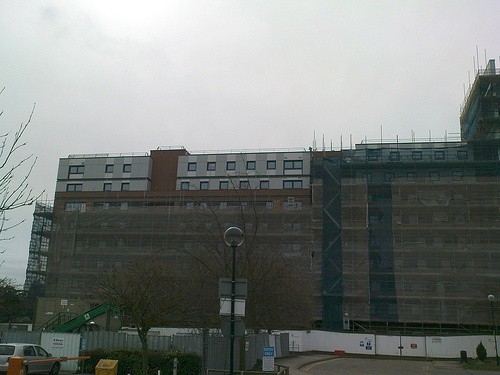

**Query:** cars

left=0, top=342, right=61, bottom=375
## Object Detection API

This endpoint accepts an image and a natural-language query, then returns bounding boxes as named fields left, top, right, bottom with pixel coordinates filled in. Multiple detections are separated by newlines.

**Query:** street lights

left=224, top=226, right=244, bottom=375
left=487, top=293, right=499, bottom=368
